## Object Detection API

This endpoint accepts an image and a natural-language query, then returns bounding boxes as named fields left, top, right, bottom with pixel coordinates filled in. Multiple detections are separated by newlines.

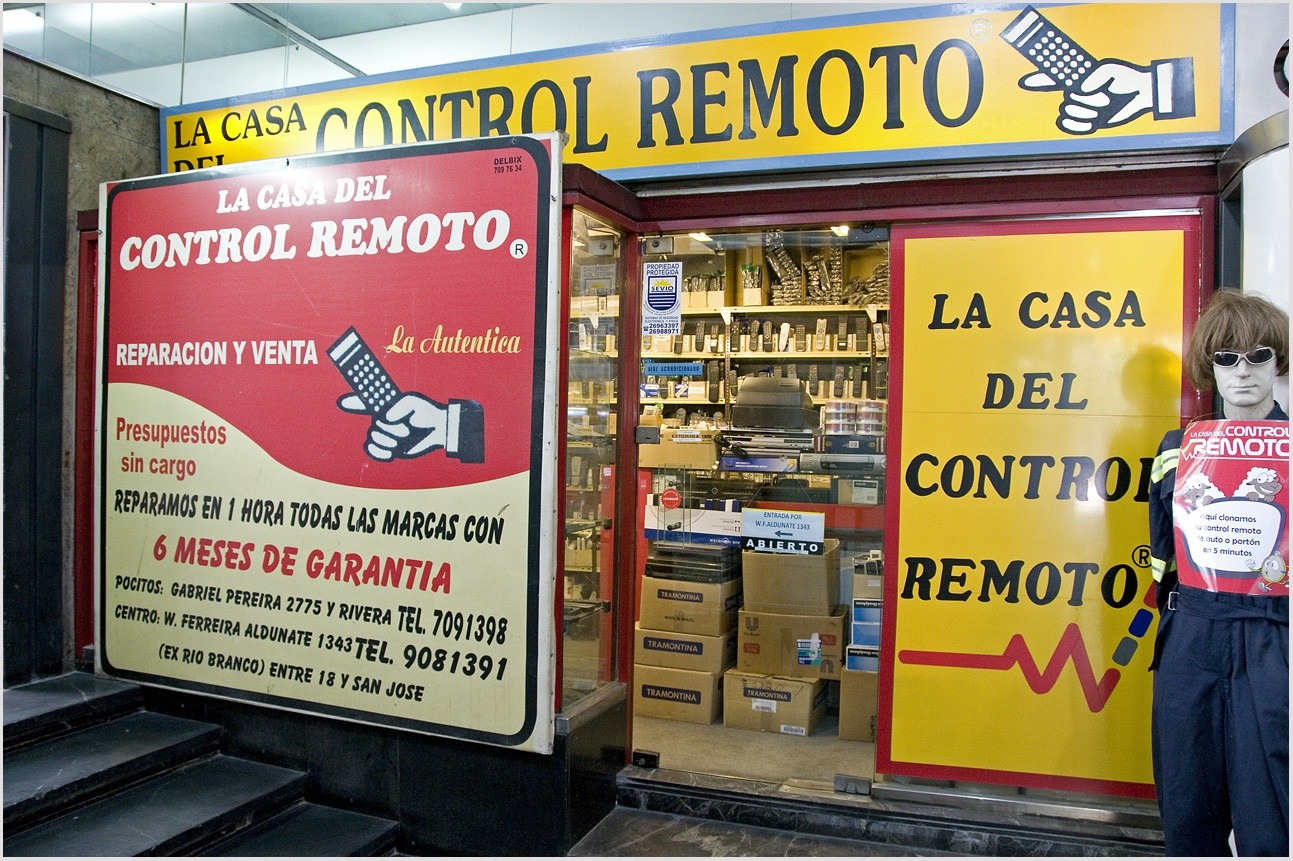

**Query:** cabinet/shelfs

left=564, top=228, right=890, bottom=641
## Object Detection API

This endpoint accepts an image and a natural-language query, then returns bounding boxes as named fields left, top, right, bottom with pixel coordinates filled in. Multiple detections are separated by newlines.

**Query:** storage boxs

left=638, top=430, right=721, bottom=471
left=741, top=537, right=840, bottom=617
left=632, top=620, right=828, bottom=736
left=736, top=603, right=849, bottom=680
left=638, top=574, right=744, bottom=637
left=836, top=573, right=884, bottom=745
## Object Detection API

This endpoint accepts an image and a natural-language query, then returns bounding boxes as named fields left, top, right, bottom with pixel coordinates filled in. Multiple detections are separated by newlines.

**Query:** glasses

left=1212, top=346, right=1280, bottom=368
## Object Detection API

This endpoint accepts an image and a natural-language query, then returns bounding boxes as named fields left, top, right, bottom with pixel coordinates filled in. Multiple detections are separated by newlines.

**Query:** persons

left=1149, top=296, right=1289, bottom=857
